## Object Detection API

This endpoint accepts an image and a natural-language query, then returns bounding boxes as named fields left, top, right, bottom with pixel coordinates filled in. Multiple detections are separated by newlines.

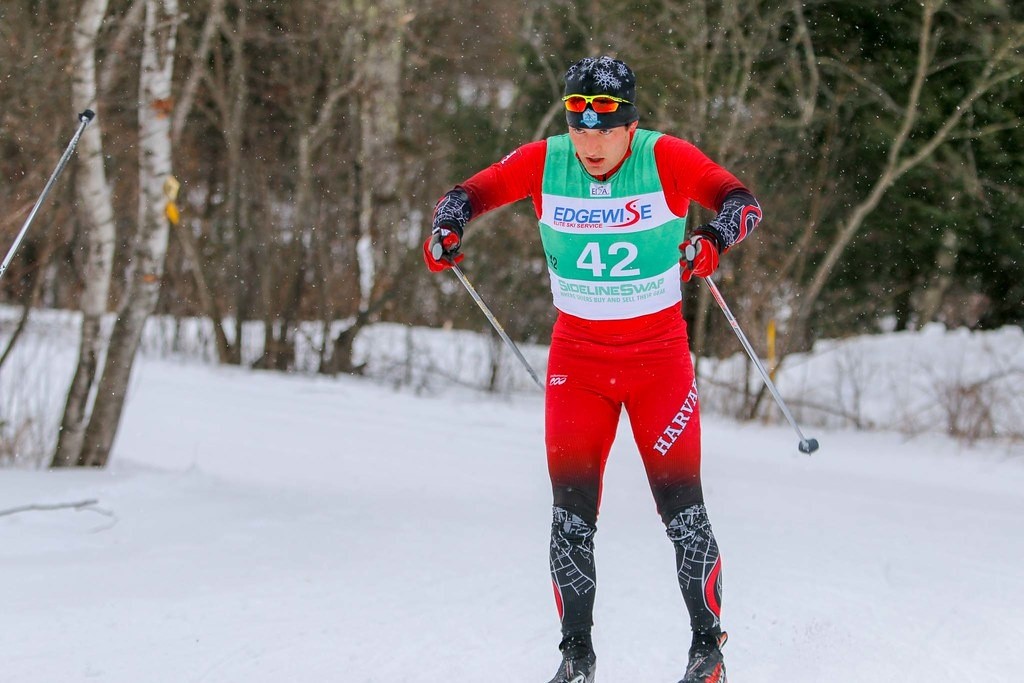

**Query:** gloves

left=423, top=230, right=465, bottom=273
left=678, top=234, right=719, bottom=281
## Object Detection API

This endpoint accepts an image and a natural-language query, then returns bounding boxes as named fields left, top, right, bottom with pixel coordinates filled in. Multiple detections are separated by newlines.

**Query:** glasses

left=562, top=94, right=632, bottom=113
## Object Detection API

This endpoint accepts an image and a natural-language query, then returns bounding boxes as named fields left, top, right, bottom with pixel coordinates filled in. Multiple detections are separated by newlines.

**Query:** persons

left=422, top=56, right=764, bottom=683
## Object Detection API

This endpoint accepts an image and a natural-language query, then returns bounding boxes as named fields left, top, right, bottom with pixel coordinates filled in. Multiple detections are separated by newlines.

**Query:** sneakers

left=679, top=631, right=727, bottom=683
left=547, top=646, right=597, bottom=683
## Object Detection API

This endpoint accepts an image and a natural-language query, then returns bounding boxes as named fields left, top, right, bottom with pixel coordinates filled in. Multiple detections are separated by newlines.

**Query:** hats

left=564, top=56, right=640, bottom=128
